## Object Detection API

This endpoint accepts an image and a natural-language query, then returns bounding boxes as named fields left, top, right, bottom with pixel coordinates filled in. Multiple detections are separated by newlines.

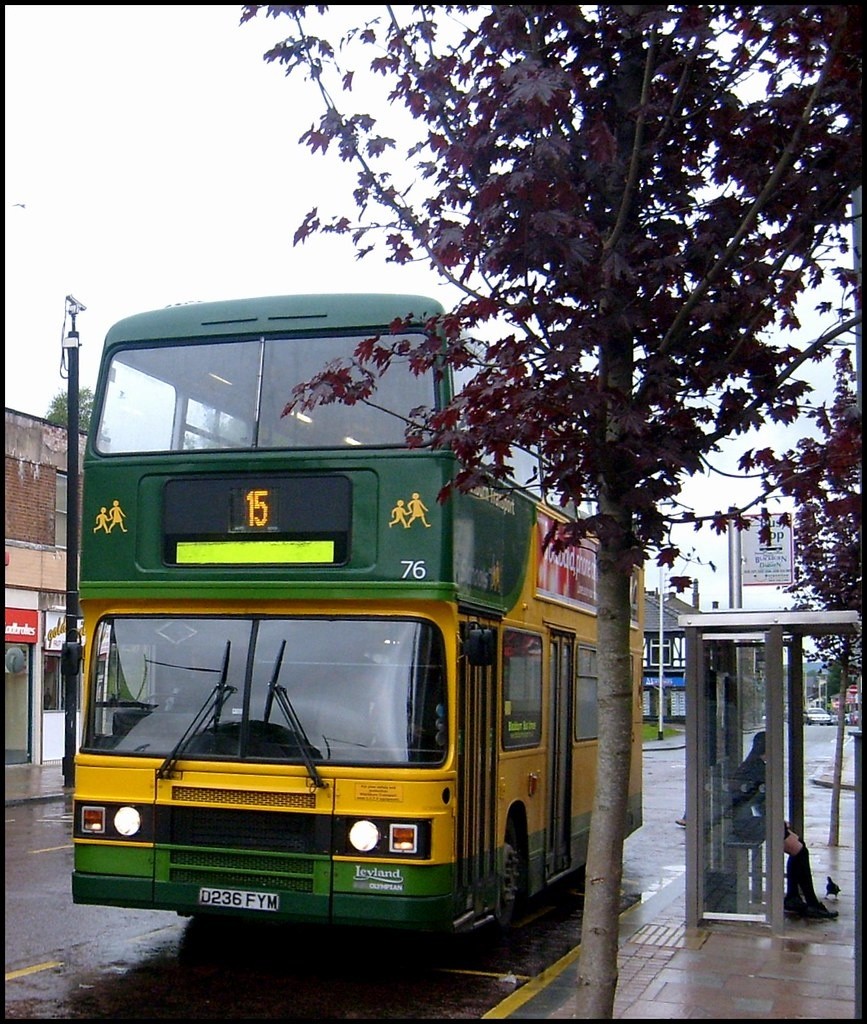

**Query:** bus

left=71, top=293, right=645, bottom=952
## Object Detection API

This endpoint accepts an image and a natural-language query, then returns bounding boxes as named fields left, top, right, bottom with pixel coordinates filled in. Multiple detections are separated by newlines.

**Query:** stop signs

left=849, top=685, right=858, bottom=694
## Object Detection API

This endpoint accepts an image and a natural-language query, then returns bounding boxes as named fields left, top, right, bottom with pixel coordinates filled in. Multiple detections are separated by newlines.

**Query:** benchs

left=723, top=819, right=789, bottom=915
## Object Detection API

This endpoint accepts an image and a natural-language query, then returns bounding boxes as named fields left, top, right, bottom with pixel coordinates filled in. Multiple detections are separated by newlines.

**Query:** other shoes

left=784, top=896, right=806, bottom=913
left=675, top=819, right=686, bottom=826
left=806, top=901, right=839, bottom=918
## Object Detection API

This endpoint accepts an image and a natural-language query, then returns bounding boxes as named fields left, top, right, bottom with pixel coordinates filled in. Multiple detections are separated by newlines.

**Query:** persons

left=728, top=732, right=839, bottom=917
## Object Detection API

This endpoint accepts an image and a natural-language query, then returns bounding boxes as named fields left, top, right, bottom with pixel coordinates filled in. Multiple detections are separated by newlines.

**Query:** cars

left=804, top=708, right=830, bottom=726
left=849, top=710, right=859, bottom=725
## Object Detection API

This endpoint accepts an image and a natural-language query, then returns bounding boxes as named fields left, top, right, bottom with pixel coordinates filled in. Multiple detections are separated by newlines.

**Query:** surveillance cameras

left=65, top=294, right=87, bottom=311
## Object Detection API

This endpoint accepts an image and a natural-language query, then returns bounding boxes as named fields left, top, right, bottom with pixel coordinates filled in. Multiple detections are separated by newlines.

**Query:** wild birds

left=824, top=876, right=841, bottom=900
